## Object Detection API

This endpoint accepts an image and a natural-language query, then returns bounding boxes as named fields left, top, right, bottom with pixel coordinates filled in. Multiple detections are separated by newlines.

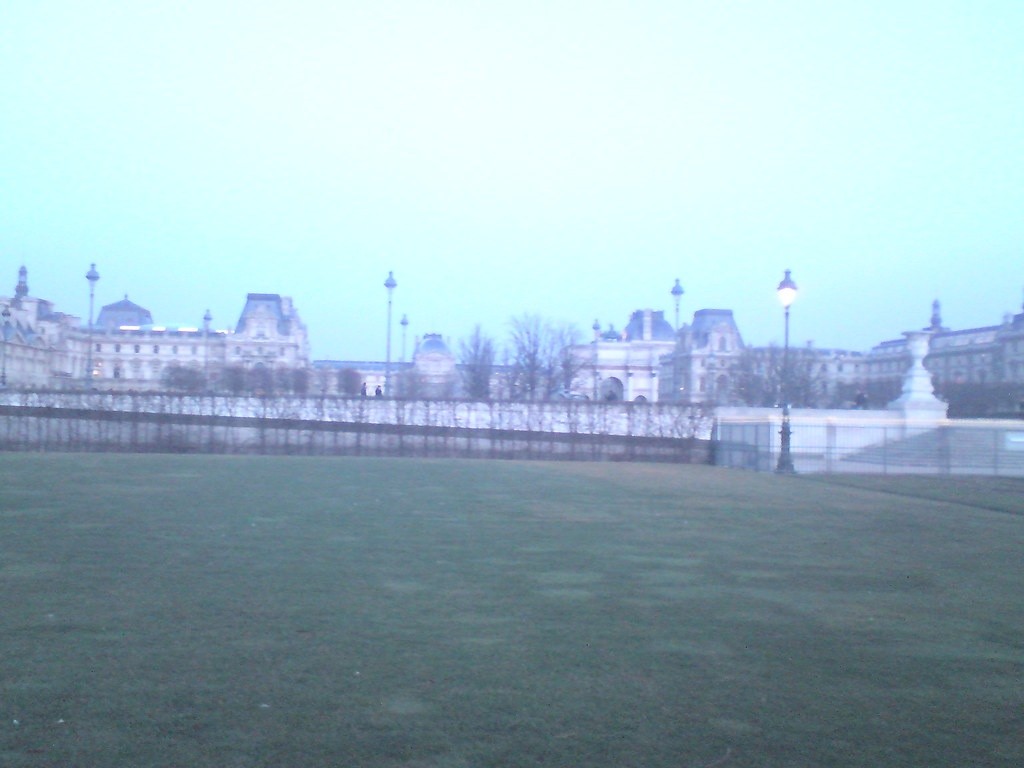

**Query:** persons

left=360, top=382, right=366, bottom=395
left=375, top=386, right=382, bottom=397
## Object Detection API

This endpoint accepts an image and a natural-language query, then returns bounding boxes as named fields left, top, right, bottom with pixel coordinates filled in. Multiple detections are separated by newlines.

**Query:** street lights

left=592, top=319, right=602, bottom=403
left=203, top=310, right=213, bottom=385
left=400, top=314, right=409, bottom=363
left=84, top=262, right=99, bottom=392
left=766, top=268, right=800, bottom=475
left=669, top=277, right=687, bottom=350
left=384, top=268, right=398, bottom=425
